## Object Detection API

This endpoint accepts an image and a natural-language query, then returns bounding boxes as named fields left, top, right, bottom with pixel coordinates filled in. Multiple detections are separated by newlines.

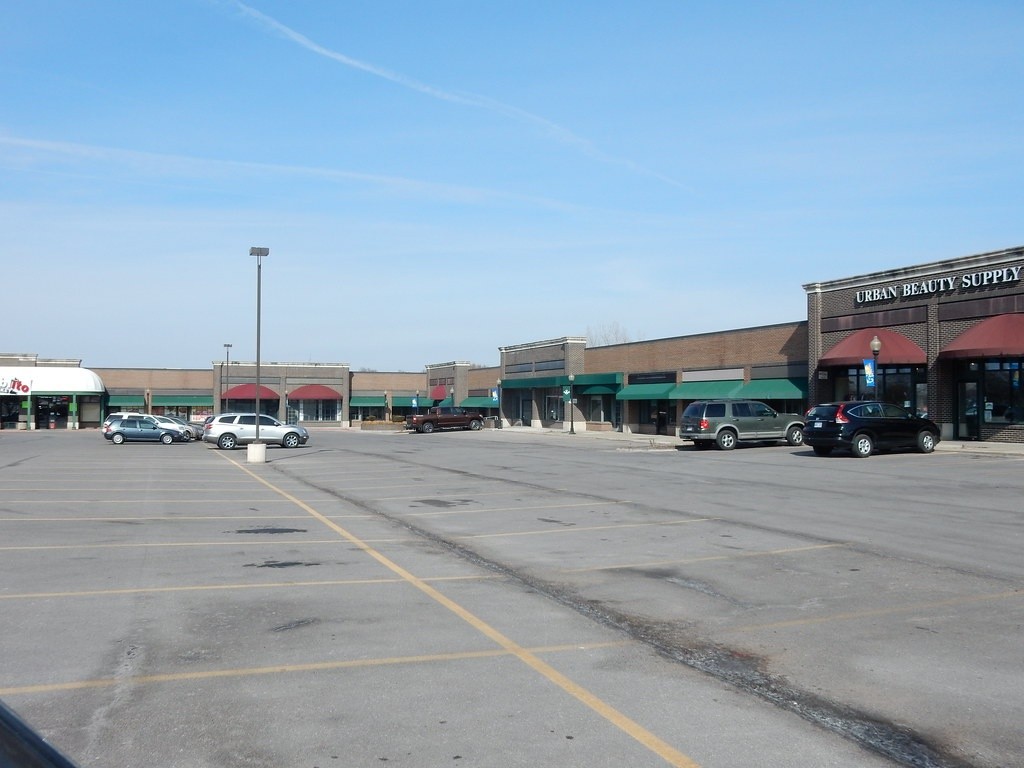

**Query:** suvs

left=801, top=400, right=940, bottom=459
left=679, top=399, right=806, bottom=452
left=100, top=411, right=194, bottom=443
left=200, top=412, right=310, bottom=450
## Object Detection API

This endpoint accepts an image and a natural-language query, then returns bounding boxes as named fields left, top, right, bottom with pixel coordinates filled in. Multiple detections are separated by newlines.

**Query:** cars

left=144, top=410, right=211, bottom=440
left=104, top=418, right=184, bottom=445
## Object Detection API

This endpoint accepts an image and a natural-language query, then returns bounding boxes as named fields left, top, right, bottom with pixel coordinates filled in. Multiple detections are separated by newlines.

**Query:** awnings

left=460, top=396, right=499, bottom=408
left=735, top=378, right=807, bottom=400
left=940, top=313, right=1024, bottom=358
left=288, top=384, right=342, bottom=400
left=616, top=384, right=676, bottom=400
left=669, top=381, right=743, bottom=400
left=106, top=395, right=214, bottom=407
left=419, top=386, right=454, bottom=408
left=350, top=396, right=386, bottom=407
left=817, top=328, right=926, bottom=368
left=221, top=383, right=281, bottom=399
left=392, top=396, right=420, bottom=407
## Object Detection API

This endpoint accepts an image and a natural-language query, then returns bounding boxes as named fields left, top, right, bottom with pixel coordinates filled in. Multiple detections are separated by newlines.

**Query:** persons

left=40, top=404, right=61, bottom=427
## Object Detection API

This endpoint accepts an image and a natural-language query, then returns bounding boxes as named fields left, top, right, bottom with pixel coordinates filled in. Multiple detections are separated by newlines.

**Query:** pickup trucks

left=404, top=405, right=484, bottom=433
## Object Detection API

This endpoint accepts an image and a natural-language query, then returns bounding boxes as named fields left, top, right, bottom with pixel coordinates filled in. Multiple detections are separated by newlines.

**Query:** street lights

left=384, top=389, right=388, bottom=420
left=495, top=377, right=502, bottom=429
left=449, top=387, right=455, bottom=407
left=284, top=390, right=288, bottom=425
left=224, top=343, right=232, bottom=413
left=870, top=335, right=882, bottom=401
left=145, top=388, right=151, bottom=413
left=415, top=389, right=420, bottom=415
left=246, top=245, right=270, bottom=444
left=568, top=373, right=576, bottom=434
